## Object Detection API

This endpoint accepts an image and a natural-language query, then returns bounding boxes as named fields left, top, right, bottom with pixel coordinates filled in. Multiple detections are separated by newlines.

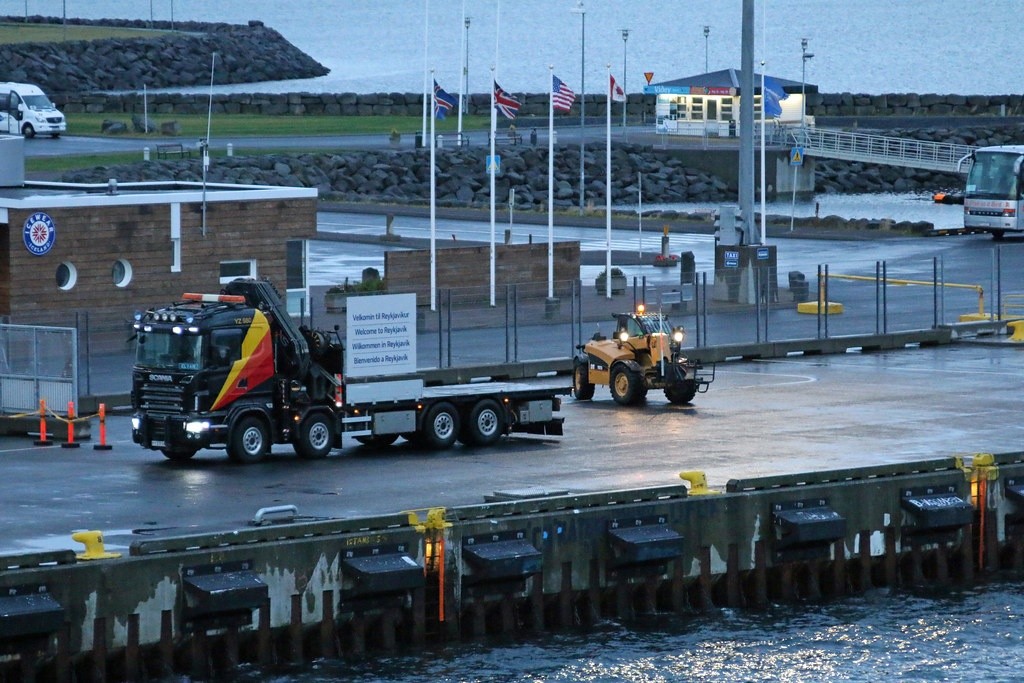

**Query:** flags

left=493, top=78, right=523, bottom=121
left=434, top=78, right=457, bottom=122
left=764, top=73, right=791, bottom=117
left=609, top=74, right=626, bottom=102
left=553, top=74, right=576, bottom=112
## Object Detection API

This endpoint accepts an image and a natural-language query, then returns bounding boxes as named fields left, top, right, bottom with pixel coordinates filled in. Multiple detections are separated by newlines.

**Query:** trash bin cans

left=199, top=137, right=208, bottom=156
left=415, top=130, right=423, bottom=148
left=729, top=119, right=737, bottom=137
left=530, top=128, right=537, bottom=145
left=679, top=251, right=696, bottom=286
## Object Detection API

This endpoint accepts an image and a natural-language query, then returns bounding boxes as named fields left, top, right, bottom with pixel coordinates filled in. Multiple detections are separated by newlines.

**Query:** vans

left=0, top=81, right=66, bottom=138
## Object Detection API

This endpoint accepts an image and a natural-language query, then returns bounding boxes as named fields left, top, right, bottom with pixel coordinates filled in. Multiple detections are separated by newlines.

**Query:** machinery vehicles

left=572, top=304, right=715, bottom=406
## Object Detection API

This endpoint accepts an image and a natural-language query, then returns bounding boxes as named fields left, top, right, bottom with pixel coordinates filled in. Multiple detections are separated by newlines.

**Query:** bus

left=957, top=144, right=1024, bottom=237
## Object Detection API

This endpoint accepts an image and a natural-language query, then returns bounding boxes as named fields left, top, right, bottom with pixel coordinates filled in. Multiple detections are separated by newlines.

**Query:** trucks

left=131, top=278, right=572, bottom=464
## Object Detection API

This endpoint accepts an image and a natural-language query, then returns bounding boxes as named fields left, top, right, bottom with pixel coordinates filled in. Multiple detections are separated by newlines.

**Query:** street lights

left=801, top=38, right=814, bottom=147
left=703, top=25, right=710, bottom=151
left=465, top=17, right=470, bottom=117
left=620, top=29, right=630, bottom=142
left=571, top=2, right=587, bottom=217
